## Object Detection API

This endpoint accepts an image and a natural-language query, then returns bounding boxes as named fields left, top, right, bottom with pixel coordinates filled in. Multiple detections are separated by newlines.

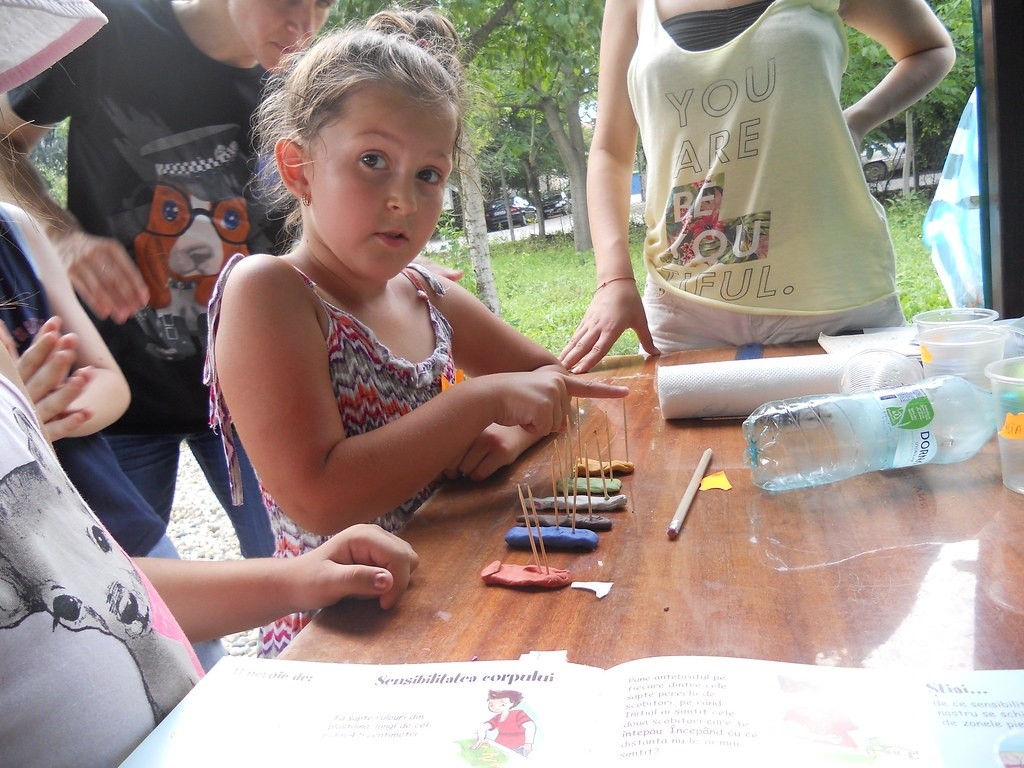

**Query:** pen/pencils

left=666, top=448, right=712, bottom=538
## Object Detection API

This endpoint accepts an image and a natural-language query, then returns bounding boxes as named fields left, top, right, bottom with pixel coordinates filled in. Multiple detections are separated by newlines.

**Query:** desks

left=286, top=336, right=1024, bottom=668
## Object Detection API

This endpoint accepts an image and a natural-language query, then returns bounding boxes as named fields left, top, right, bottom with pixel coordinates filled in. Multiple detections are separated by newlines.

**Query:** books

left=118, top=653, right=1023, bottom=768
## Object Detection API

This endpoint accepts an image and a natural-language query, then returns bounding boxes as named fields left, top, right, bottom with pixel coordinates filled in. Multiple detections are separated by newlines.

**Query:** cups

left=983, top=355, right=1024, bottom=494
left=914, top=325, right=1008, bottom=394
left=836, top=349, right=924, bottom=398
left=911, top=307, right=1000, bottom=332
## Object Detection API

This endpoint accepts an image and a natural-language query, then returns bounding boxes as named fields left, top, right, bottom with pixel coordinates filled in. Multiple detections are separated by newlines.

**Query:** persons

left=0, top=377, right=421, bottom=768
left=0, top=0, right=338, bottom=559
left=554, top=1, right=958, bottom=374
left=203, top=10, right=629, bottom=660
left=0, top=0, right=228, bottom=675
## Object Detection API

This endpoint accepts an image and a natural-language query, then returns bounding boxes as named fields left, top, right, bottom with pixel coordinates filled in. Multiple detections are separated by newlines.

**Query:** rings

left=99, top=264, right=107, bottom=277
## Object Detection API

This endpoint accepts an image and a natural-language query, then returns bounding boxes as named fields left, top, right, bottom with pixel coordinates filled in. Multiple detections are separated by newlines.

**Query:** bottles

left=742, top=375, right=1022, bottom=492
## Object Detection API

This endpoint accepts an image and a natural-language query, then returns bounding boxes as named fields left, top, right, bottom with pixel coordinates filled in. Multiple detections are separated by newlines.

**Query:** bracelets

left=593, top=277, right=636, bottom=294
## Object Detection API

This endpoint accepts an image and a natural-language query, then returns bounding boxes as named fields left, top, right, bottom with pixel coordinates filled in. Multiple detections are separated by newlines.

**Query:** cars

left=858, top=140, right=907, bottom=183
left=538, top=189, right=573, bottom=219
left=484, top=195, right=537, bottom=231
